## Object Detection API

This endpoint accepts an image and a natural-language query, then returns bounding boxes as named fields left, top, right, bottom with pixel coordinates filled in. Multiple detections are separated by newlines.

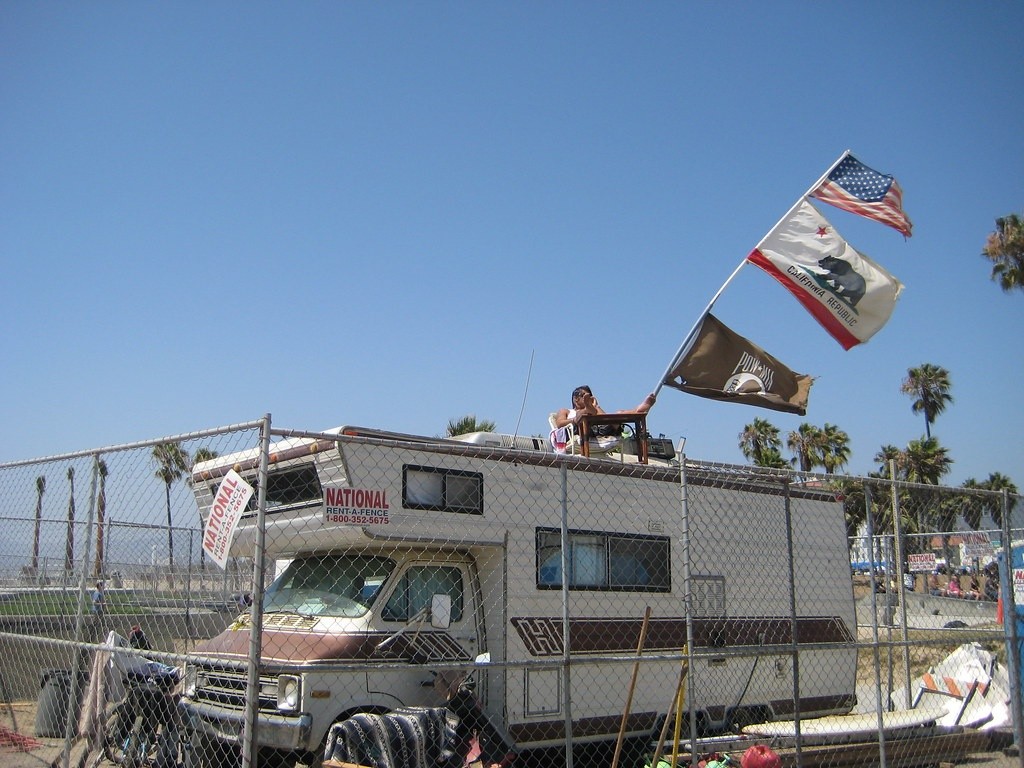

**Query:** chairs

left=547, top=411, right=630, bottom=462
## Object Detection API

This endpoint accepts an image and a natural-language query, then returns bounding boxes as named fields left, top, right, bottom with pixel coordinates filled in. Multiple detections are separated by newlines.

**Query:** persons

left=873, top=561, right=1000, bottom=604
left=92, top=582, right=104, bottom=641
left=556, top=385, right=656, bottom=435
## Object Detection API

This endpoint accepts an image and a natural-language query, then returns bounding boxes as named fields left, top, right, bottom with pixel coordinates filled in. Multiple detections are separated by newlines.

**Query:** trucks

left=177, top=424, right=859, bottom=768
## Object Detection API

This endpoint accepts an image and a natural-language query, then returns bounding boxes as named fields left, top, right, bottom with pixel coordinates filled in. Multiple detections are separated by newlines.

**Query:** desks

left=574, top=411, right=649, bottom=464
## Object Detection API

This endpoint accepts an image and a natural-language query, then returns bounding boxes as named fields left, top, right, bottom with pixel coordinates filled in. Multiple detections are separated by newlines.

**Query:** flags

left=665, top=312, right=815, bottom=416
left=809, top=155, right=913, bottom=238
left=747, top=197, right=906, bottom=351
